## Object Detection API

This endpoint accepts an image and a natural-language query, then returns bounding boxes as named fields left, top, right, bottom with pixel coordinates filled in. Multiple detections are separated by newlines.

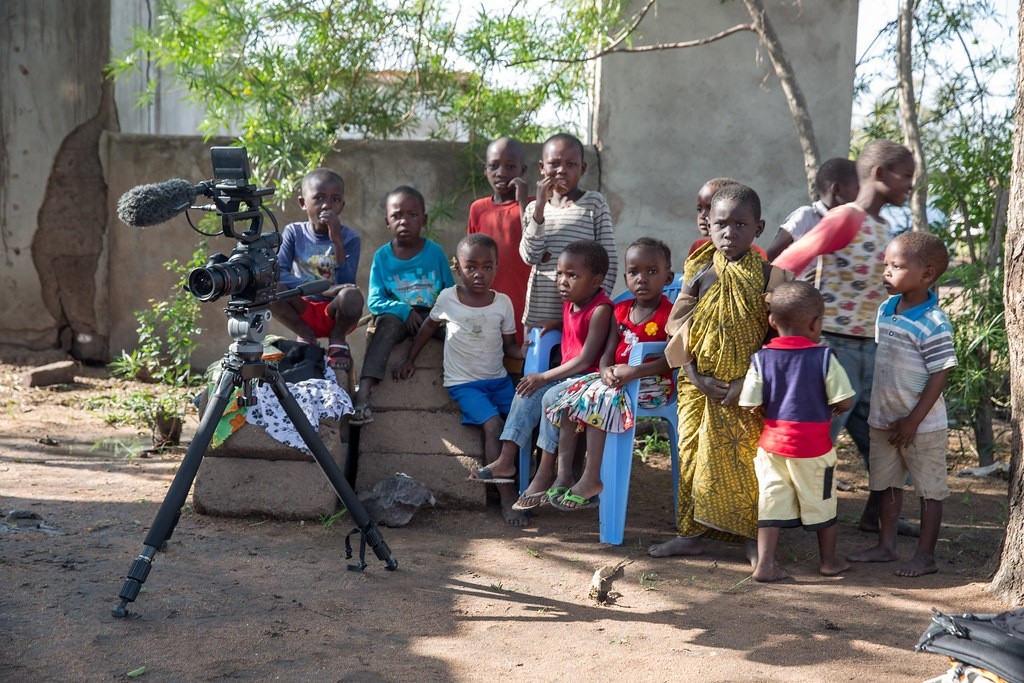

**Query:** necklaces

left=632, top=300, right=663, bottom=326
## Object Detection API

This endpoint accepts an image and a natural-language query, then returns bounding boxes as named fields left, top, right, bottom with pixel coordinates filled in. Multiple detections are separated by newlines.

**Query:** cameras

left=188, top=231, right=283, bottom=301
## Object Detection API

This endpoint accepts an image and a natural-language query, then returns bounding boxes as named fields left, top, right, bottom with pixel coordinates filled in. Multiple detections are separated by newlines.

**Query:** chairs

left=517, top=272, right=684, bottom=546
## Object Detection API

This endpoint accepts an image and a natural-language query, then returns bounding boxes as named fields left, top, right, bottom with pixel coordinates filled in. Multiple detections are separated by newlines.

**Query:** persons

left=465, top=239, right=615, bottom=512
left=843, top=231, right=959, bottom=577
left=539, top=237, right=675, bottom=511
left=763, top=157, right=860, bottom=265
left=349, top=185, right=456, bottom=425
left=518, top=133, right=618, bottom=484
left=738, top=280, right=856, bottom=582
left=687, top=177, right=768, bottom=262
left=391, top=233, right=534, bottom=528
left=647, top=183, right=796, bottom=570
left=466, top=137, right=536, bottom=391
left=770, top=138, right=921, bottom=538
left=267, top=167, right=364, bottom=370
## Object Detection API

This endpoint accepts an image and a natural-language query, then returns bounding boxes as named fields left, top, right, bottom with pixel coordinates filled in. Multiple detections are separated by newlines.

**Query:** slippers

left=465, top=468, right=515, bottom=484
left=540, top=487, right=600, bottom=512
left=512, top=491, right=547, bottom=511
left=349, top=404, right=375, bottom=425
left=329, top=341, right=353, bottom=369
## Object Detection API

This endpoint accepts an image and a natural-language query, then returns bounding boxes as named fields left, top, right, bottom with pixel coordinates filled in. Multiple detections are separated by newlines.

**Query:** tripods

left=111, top=286, right=400, bottom=618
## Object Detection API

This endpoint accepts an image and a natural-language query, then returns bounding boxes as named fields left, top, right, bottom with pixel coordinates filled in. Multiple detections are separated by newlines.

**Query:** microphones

left=117, top=178, right=214, bottom=227
